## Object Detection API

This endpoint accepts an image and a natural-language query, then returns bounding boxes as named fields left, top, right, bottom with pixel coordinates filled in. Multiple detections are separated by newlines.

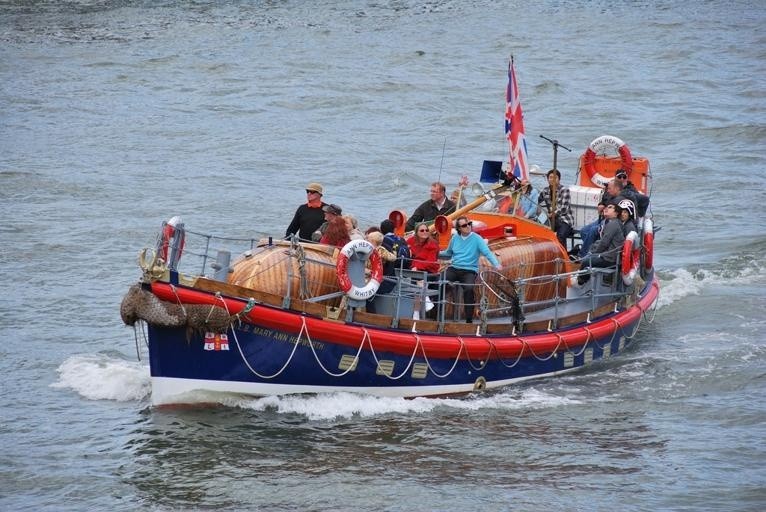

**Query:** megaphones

left=479, top=159, right=505, bottom=185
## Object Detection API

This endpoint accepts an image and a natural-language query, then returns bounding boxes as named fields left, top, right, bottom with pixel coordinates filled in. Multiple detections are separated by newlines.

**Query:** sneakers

left=569, top=254, right=581, bottom=260
left=571, top=277, right=586, bottom=290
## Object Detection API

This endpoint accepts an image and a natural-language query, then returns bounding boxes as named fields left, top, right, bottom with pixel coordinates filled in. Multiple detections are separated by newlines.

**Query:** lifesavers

left=622, top=231, right=641, bottom=285
left=585, top=135, right=631, bottom=189
left=157, top=216, right=185, bottom=269
left=336, top=240, right=383, bottom=300
left=644, top=218, right=653, bottom=272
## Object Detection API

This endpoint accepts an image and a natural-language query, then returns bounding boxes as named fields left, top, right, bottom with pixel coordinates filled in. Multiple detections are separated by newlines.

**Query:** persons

left=285, top=183, right=330, bottom=243
left=405, top=223, right=440, bottom=321
left=515, top=184, right=538, bottom=221
left=571, top=168, right=650, bottom=288
left=405, top=182, right=456, bottom=232
left=451, top=189, right=467, bottom=208
left=539, top=170, right=574, bottom=252
left=434, top=217, right=503, bottom=323
left=312, top=205, right=410, bottom=313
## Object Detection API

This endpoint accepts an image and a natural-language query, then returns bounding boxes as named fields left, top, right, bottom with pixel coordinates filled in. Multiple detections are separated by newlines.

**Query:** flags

left=505, top=54, right=529, bottom=183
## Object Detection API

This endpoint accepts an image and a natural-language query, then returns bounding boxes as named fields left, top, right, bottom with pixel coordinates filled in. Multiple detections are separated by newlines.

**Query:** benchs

left=566, top=225, right=584, bottom=250
left=585, top=266, right=617, bottom=306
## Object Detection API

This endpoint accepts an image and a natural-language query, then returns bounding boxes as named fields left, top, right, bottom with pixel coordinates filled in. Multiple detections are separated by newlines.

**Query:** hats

left=305, top=183, right=323, bottom=196
left=618, top=200, right=637, bottom=220
left=323, top=204, right=342, bottom=216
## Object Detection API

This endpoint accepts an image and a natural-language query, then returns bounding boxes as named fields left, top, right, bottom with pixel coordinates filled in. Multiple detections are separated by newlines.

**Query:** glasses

left=618, top=175, right=626, bottom=179
left=421, top=229, right=429, bottom=232
left=307, top=190, right=317, bottom=194
left=615, top=169, right=625, bottom=176
left=460, top=222, right=472, bottom=228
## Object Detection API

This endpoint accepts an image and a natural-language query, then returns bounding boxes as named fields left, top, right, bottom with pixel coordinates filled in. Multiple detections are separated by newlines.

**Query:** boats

left=120, top=136, right=664, bottom=404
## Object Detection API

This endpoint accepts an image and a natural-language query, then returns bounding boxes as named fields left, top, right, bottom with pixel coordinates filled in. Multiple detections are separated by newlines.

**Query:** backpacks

left=385, top=236, right=410, bottom=266
